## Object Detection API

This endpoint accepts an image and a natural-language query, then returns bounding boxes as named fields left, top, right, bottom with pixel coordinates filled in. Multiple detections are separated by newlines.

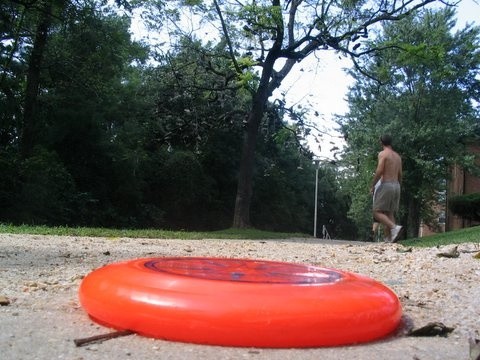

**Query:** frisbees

left=77, top=257, right=401, bottom=348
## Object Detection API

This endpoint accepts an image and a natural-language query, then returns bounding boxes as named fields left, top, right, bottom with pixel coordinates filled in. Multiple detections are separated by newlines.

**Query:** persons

left=369, top=175, right=384, bottom=241
left=370, top=133, right=404, bottom=244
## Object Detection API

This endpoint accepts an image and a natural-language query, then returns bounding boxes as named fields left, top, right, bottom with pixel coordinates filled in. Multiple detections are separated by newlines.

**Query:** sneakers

left=384, top=237, right=390, bottom=243
left=369, top=231, right=375, bottom=242
left=390, top=225, right=404, bottom=243
